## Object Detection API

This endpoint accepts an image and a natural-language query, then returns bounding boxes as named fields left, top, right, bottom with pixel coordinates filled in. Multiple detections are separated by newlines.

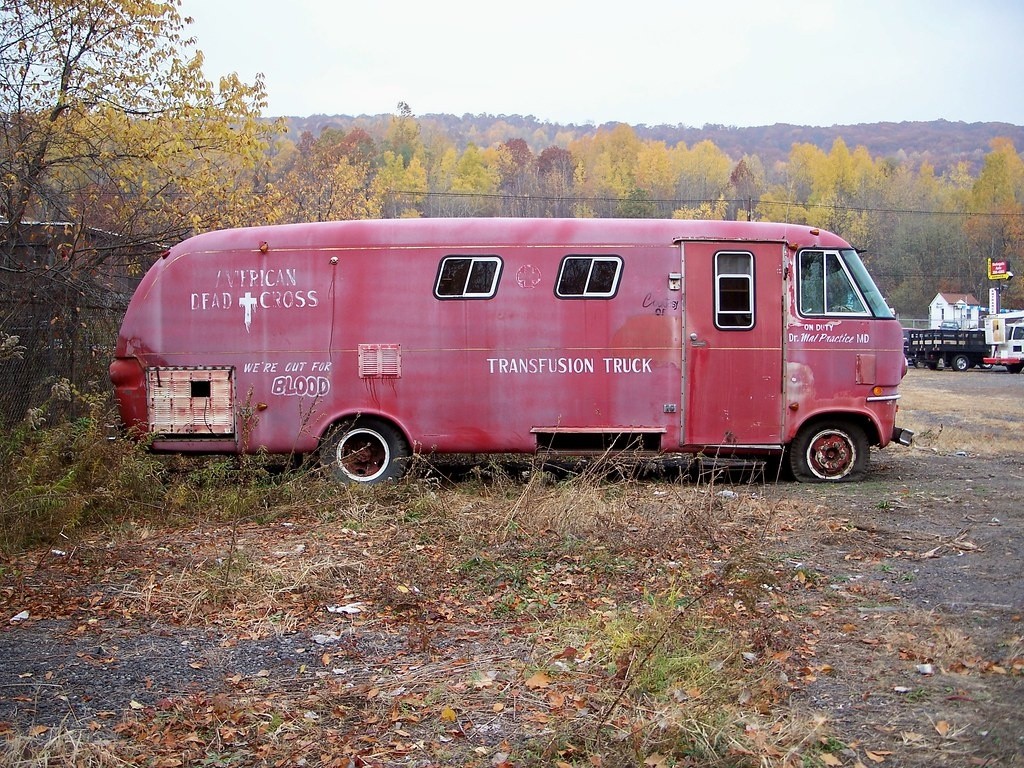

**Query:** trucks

left=901, top=309, right=1024, bottom=374
left=108, top=217, right=916, bottom=483
